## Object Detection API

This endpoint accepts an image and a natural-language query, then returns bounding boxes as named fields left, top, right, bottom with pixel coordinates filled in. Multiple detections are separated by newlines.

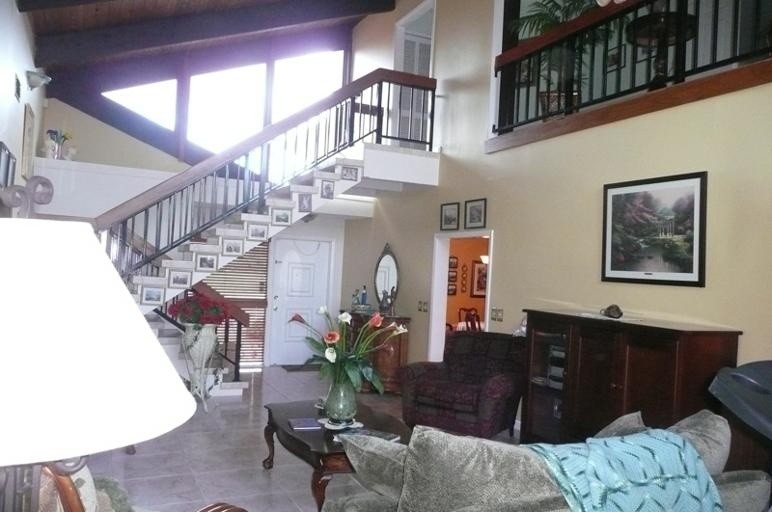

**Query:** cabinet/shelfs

left=524, top=312, right=573, bottom=444
left=337, top=309, right=411, bottom=394
left=578, top=318, right=681, bottom=429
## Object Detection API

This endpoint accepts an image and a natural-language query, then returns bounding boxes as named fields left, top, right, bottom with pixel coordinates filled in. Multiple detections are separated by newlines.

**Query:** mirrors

left=373, top=245, right=401, bottom=313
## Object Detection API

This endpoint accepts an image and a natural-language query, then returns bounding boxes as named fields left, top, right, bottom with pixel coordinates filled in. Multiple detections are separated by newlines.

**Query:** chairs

left=459, top=307, right=478, bottom=323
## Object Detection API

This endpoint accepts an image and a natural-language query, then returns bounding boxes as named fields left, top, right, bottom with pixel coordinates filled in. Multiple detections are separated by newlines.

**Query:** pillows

left=336, top=430, right=407, bottom=497
left=398, top=408, right=729, bottom=511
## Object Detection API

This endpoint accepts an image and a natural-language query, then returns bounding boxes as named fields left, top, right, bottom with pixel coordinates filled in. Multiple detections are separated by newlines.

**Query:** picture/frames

left=464, top=198, right=487, bottom=232
left=598, top=172, right=707, bottom=287
left=449, top=271, right=457, bottom=282
left=470, top=260, right=486, bottom=297
left=21, top=103, right=35, bottom=181
left=450, top=256, right=458, bottom=268
left=441, top=201, right=460, bottom=233
left=448, top=285, right=457, bottom=296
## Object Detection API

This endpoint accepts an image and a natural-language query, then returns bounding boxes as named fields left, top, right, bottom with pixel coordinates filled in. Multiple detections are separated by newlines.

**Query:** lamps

left=26, top=66, right=52, bottom=90
left=7, top=218, right=198, bottom=512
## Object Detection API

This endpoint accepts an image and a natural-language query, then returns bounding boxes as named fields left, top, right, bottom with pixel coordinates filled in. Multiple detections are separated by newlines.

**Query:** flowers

left=168, top=292, right=227, bottom=323
left=46, top=127, right=73, bottom=142
left=289, top=304, right=407, bottom=396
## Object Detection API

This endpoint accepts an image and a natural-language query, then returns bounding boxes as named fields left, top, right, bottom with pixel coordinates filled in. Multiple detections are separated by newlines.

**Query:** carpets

left=97, top=477, right=132, bottom=512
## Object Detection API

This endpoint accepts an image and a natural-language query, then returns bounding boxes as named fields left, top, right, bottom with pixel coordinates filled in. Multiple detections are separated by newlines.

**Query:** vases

left=324, top=383, right=358, bottom=421
left=50, top=141, right=63, bottom=159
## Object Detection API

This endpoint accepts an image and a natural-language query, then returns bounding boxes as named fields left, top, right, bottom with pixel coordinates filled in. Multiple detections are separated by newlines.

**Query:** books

left=289, top=416, right=321, bottom=432
left=332, top=426, right=402, bottom=449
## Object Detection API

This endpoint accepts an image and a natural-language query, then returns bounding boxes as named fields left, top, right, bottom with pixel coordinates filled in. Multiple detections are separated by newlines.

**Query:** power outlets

left=498, top=308, right=503, bottom=320
left=491, top=307, right=498, bottom=320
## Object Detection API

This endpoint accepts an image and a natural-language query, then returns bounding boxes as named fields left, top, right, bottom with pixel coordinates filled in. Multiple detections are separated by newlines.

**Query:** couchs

left=396, top=330, right=527, bottom=439
left=322, top=470, right=771, bottom=512
